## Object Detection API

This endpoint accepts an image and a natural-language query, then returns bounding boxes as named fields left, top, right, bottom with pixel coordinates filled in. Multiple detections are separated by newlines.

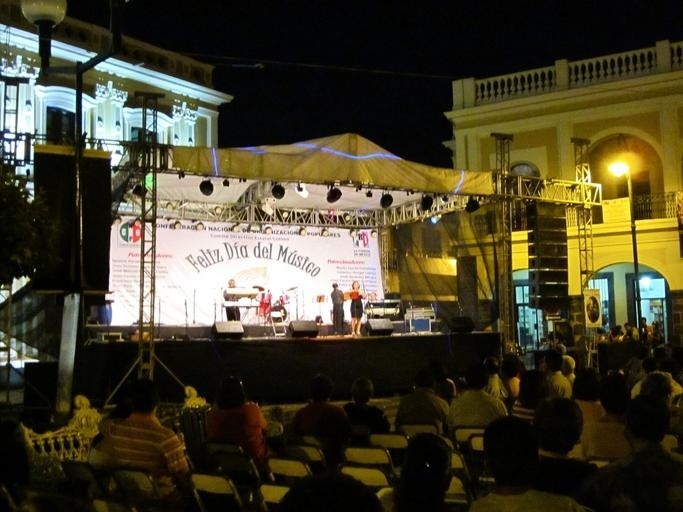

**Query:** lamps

left=380, top=190, right=394, bottom=208
left=326, top=186, right=343, bottom=203
left=464, top=197, right=481, bottom=213
left=269, top=185, right=287, bottom=200
left=198, top=177, right=218, bottom=197
left=431, top=213, right=443, bottom=223
left=419, top=195, right=434, bottom=213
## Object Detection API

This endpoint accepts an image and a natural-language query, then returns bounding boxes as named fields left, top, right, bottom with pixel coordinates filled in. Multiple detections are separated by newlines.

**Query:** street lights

left=18, top=0, right=122, bottom=347
left=602, top=130, right=647, bottom=342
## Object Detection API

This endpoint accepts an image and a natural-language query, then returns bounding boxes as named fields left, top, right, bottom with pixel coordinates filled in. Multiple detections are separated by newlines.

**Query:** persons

left=331, top=283, right=345, bottom=336
left=349, top=280, right=366, bottom=337
left=111, top=379, right=189, bottom=491
left=224, top=279, right=240, bottom=323
left=204, top=319, right=682, bottom=511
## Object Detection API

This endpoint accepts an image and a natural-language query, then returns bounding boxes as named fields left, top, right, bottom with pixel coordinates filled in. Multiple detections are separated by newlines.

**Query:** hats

left=562, top=355, right=576, bottom=370
